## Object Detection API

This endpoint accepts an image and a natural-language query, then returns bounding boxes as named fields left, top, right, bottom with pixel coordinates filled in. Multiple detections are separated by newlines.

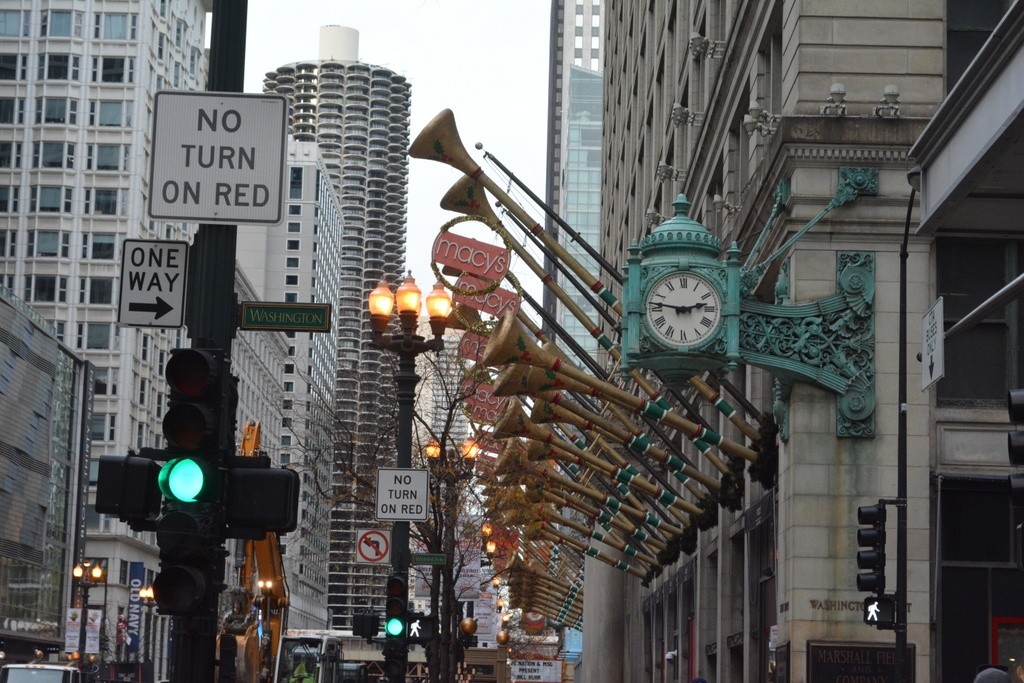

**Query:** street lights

left=369, top=269, right=454, bottom=682
left=894, top=168, right=923, bottom=681
left=427, top=433, right=482, bottom=683
left=73, top=559, right=103, bottom=663
left=138, top=585, right=155, bottom=683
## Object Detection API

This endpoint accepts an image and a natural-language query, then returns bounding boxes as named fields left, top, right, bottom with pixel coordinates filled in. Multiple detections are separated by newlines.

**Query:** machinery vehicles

left=216, top=417, right=344, bottom=683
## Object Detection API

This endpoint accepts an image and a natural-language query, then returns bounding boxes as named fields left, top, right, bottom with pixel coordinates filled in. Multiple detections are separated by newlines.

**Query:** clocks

left=618, top=166, right=883, bottom=439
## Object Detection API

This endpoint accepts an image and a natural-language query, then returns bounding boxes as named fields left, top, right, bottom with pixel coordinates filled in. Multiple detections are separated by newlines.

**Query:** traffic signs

left=920, top=296, right=945, bottom=392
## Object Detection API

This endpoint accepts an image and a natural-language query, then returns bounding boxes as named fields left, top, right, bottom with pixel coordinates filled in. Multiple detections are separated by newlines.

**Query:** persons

left=290, top=654, right=318, bottom=682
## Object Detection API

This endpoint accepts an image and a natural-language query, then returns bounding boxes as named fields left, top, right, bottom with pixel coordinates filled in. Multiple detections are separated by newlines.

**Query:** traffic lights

left=863, top=597, right=896, bottom=624
left=384, top=573, right=409, bottom=642
left=409, top=616, right=434, bottom=641
left=146, top=512, right=219, bottom=617
left=856, top=496, right=889, bottom=593
left=1006, top=389, right=1024, bottom=514
left=158, top=350, right=228, bottom=504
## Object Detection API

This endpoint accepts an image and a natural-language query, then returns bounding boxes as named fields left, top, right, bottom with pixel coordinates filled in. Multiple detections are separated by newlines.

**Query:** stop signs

left=116, top=238, right=190, bottom=332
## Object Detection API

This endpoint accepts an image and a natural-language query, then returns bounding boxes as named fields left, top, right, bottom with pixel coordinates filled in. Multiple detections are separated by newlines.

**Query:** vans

left=0, top=663, right=83, bottom=683
